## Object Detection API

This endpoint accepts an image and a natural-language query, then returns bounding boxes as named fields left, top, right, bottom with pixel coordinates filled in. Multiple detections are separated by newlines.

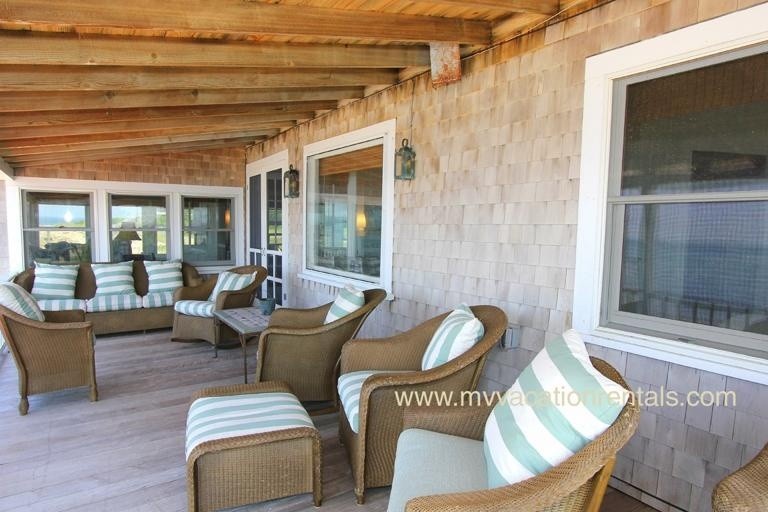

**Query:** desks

left=211, top=306, right=285, bottom=384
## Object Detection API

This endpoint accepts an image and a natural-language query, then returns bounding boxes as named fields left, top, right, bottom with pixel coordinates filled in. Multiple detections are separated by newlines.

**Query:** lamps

left=396, top=137, right=415, bottom=180
left=284, top=164, right=299, bottom=199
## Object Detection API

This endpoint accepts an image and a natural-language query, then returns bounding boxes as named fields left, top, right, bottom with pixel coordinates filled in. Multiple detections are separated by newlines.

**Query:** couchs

left=12, top=262, right=204, bottom=335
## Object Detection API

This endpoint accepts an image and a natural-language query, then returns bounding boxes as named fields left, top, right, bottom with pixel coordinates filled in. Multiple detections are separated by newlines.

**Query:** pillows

left=484, top=327, right=631, bottom=489
left=323, top=284, right=365, bottom=325
left=0, top=281, right=45, bottom=322
left=421, top=303, right=485, bottom=371
left=30, top=260, right=80, bottom=300
left=91, top=260, right=137, bottom=296
left=208, top=271, right=257, bottom=302
left=143, top=259, right=184, bottom=292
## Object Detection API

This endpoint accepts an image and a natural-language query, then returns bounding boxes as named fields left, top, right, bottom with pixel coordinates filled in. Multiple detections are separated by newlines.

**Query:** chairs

left=0, top=304, right=97, bottom=415
left=171, top=265, right=268, bottom=347
left=387, top=357, right=640, bottom=512
left=337, top=305, right=508, bottom=504
left=711, top=442, right=768, bottom=512
left=255, top=289, right=387, bottom=416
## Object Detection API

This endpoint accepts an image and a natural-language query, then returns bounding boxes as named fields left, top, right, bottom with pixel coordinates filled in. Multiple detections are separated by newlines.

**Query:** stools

left=185, top=381, right=323, bottom=511
